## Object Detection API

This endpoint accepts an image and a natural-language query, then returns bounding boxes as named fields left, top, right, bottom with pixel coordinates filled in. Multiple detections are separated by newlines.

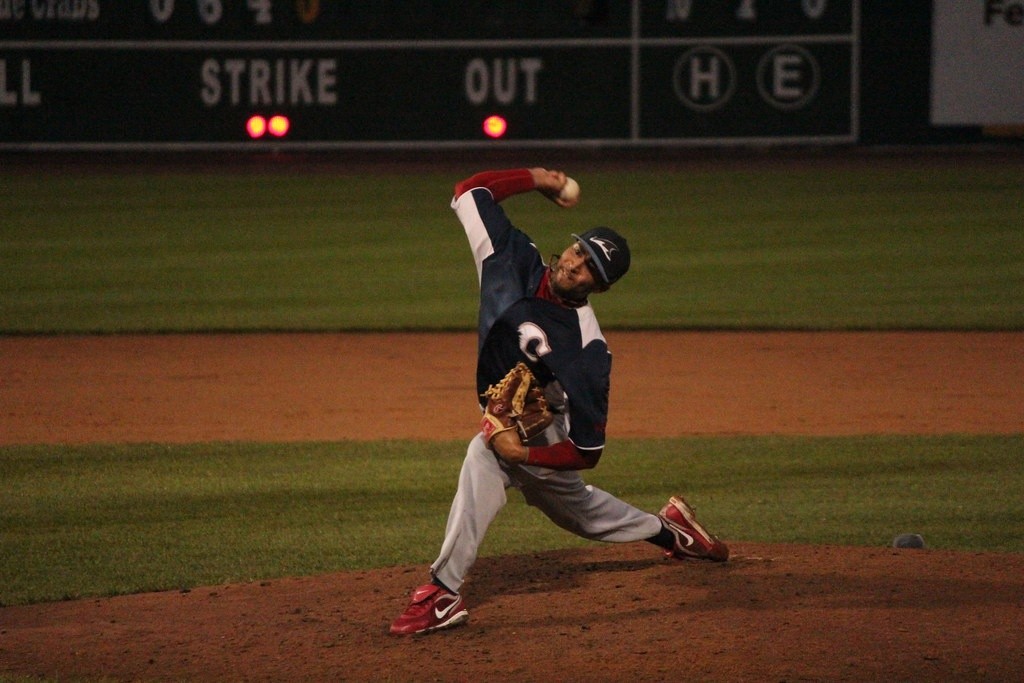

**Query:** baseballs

left=558, top=176, right=580, bottom=201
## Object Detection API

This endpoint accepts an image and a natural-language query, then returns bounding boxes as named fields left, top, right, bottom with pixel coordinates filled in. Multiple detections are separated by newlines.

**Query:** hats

left=571, top=226, right=630, bottom=286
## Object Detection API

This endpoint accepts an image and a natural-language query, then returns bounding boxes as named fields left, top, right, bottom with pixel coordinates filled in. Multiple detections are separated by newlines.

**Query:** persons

left=390, top=167, right=734, bottom=639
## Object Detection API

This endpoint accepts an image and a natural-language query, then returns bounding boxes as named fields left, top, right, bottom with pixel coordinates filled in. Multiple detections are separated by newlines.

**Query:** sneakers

left=389, top=582, right=468, bottom=637
left=655, top=496, right=728, bottom=563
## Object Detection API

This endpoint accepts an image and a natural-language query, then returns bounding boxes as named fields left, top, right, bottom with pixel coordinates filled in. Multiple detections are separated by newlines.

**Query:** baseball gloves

left=478, top=362, right=555, bottom=450
